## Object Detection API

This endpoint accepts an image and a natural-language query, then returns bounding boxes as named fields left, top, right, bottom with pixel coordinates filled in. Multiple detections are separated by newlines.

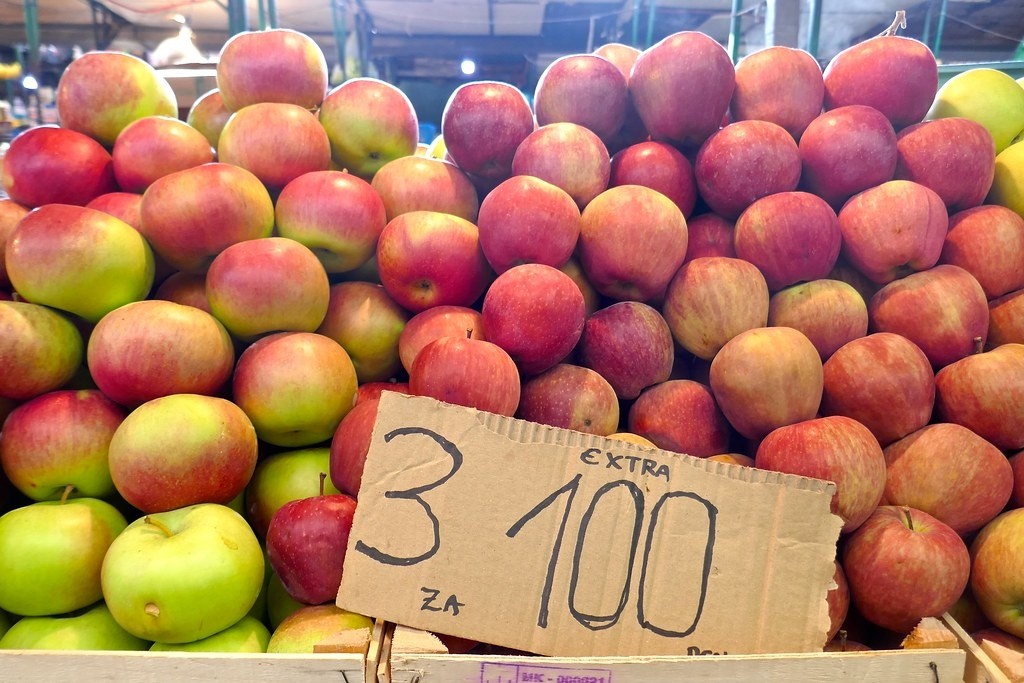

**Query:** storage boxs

left=0, top=611, right=1014, bottom=683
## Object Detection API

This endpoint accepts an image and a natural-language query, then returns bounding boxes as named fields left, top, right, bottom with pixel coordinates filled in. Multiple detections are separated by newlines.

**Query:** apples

left=1, top=30, right=1024, bottom=654
